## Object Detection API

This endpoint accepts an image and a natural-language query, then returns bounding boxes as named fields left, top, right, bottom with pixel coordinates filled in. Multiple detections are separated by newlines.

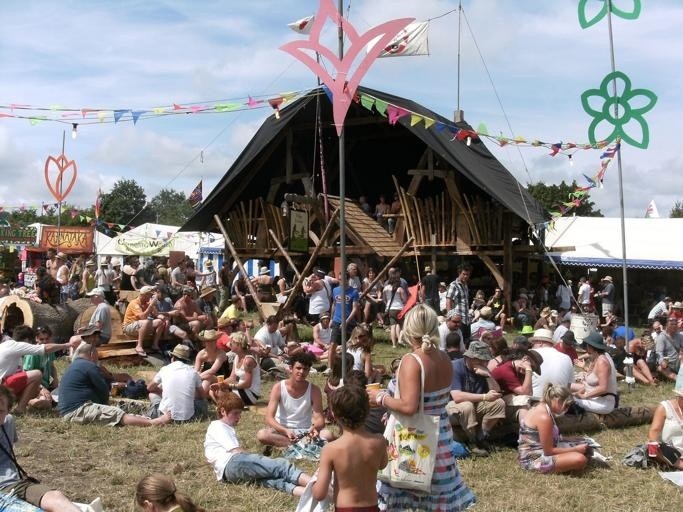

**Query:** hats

left=113, top=259, right=121, bottom=266
left=145, top=260, right=155, bottom=268
left=140, top=286, right=156, bottom=295
left=671, top=302, right=683, bottom=309
left=81, top=324, right=100, bottom=338
left=259, top=266, right=271, bottom=275
left=86, top=260, right=94, bottom=266
left=601, top=276, right=613, bottom=283
left=462, top=340, right=493, bottom=361
left=560, top=331, right=577, bottom=345
left=528, top=329, right=557, bottom=345
left=440, top=282, right=446, bottom=287
left=169, top=344, right=190, bottom=361
left=519, top=294, right=528, bottom=299
left=582, top=330, right=608, bottom=350
left=200, top=286, right=217, bottom=298
left=319, top=312, right=330, bottom=321
left=101, top=261, right=108, bottom=265
left=551, top=310, right=559, bottom=317
left=196, top=329, right=223, bottom=342
left=518, top=326, right=535, bottom=334
left=313, top=266, right=326, bottom=276
left=525, top=349, right=544, bottom=375
left=176, top=255, right=185, bottom=264
left=604, top=311, right=610, bottom=315
left=54, top=252, right=66, bottom=260
left=540, top=307, right=551, bottom=317
left=86, top=287, right=106, bottom=298
left=425, top=267, right=431, bottom=272
left=204, top=258, right=213, bottom=267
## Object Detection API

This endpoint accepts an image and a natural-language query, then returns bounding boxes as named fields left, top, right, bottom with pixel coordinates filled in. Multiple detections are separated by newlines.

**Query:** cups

left=217, top=375, right=224, bottom=383
left=365, top=383, right=380, bottom=410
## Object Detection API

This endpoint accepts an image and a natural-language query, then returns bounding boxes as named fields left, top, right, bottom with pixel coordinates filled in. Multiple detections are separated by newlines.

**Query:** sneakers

left=464, top=441, right=486, bottom=457
left=482, top=436, right=500, bottom=450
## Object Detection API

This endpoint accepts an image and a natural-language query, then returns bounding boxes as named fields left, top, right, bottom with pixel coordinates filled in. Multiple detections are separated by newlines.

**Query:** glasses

left=290, top=433, right=310, bottom=439
left=496, top=291, right=499, bottom=292
left=451, top=320, right=461, bottom=323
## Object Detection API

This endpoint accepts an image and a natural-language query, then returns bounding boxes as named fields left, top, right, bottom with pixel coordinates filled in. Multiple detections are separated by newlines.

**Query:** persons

left=445, top=332, right=464, bottom=360
left=446, top=265, right=471, bottom=341
left=69, top=287, right=112, bottom=357
left=563, top=305, right=578, bottom=321
left=471, top=306, right=495, bottom=341
left=172, top=286, right=210, bottom=338
left=110, top=261, right=122, bottom=290
left=324, top=352, right=355, bottom=419
left=105, top=255, right=113, bottom=269
left=309, top=266, right=338, bottom=287
left=152, top=255, right=169, bottom=285
left=217, top=295, right=247, bottom=335
left=45, top=247, right=58, bottom=278
left=359, top=196, right=369, bottom=211
left=311, top=384, right=389, bottom=512
left=218, top=261, right=229, bottom=313
left=231, top=271, right=258, bottom=314
left=94, top=260, right=112, bottom=302
left=347, top=262, right=362, bottom=321
left=278, top=309, right=312, bottom=344
left=554, top=331, right=578, bottom=366
left=511, top=293, right=533, bottom=326
left=534, top=308, right=550, bottom=330
left=646, top=320, right=666, bottom=361
left=648, top=296, right=672, bottom=326
left=556, top=279, right=573, bottom=311
left=384, top=270, right=404, bottom=349
left=552, top=317, right=572, bottom=343
left=344, top=369, right=387, bottom=433
left=122, top=286, right=164, bottom=357
left=256, top=353, right=334, bottom=446
left=586, top=280, right=595, bottom=309
left=185, top=260, right=214, bottom=292
left=54, top=251, right=69, bottom=303
left=421, top=265, right=440, bottom=314
left=361, top=267, right=383, bottom=322
left=373, top=304, right=477, bottom=512
left=82, top=259, right=94, bottom=297
left=528, top=329, right=575, bottom=398
left=487, top=287, right=506, bottom=330
left=471, top=290, right=486, bottom=311
left=648, top=376, right=683, bottom=472
left=491, top=349, right=544, bottom=405
left=254, top=315, right=289, bottom=372
left=195, top=286, right=219, bottom=329
left=438, top=281, right=447, bottom=315
left=149, top=284, right=196, bottom=343
left=446, top=341, right=506, bottom=456
left=517, top=382, right=592, bottom=474
left=574, top=347, right=658, bottom=387
left=573, top=330, right=618, bottom=414
left=169, top=258, right=187, bottom=285
left=547, top=309, right=560, bottom=326
left=517, top=326, right=535, bottom=339
left=657, top=318, right=683, bottom=379
left=375, top=196, right=390, bottom=215
left=594, top=276, right=614, bottom=323
left=256, top=266, right=275, bottom=303
left=0, top=384, right=83, bottom=512
left=577, top=277, right=591, bottom=312
left=133, top=472, right=206, bottom=512
left=35, top=266, right=61, bottom=308
left=481, top=331, right=532, bottom=371
left=303, top=268, right=332, bottom=325
left=58, top=343, right=172, bottom=427
left=209, top=332, right=261, bottom=405
left=384, top=268, right=408, bottom=304
left=200, top=259, right=216, bottom=289
left=22, top=324, right=59, bottom=390
left=0, top=326, right=76, bottom=415
left=599, top=309, right=622, bottom=330
left=194, top=329, right=230, bottom=394
left=322, top=271, right=359, bottom=376
left=203, top=393, right=311, bottom=497
left=136, top=260, right=154, bottom=289
left=346, top=322, right=385, bottom=382
left=121, top=257, right=140, bottom=291
left=272, top=271, right=292, bottom=303
left=391, top=193, right=401, bottom=214
left=68, top=254, right=86, bottom=286
left=147, top=344, right=203, bottom=423
left=670, top=300, right=683, bottom=327
left=312, top=310, right=332, bottom=351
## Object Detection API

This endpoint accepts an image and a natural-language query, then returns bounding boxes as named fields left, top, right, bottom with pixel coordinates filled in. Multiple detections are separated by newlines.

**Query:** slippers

left=135, top=349, right=147, bottom=358
left=151, top=348, right=162, bottom=354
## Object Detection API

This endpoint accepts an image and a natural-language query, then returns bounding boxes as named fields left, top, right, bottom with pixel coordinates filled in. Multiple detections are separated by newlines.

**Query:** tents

left=511, top=215, right=683, bottom=272
left=97, top=222, right=180, bottom=260
left=198, top=236, right=279, bottom=274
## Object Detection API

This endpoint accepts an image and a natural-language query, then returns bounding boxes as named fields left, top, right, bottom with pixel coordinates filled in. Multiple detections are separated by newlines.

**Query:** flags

left=287, top=15, right=315, bottom=35
left=367, top=20, right=430, bottom=58
left=350, top=89, right=619, bottom=208
left=189, top=179, right=203, bottom=207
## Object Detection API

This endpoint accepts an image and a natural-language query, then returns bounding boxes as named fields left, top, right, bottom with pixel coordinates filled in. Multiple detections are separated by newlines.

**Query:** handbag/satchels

left=375, top=353, right=440, bottom=494
left=383, top=286, right=398, bottom=325
left=0, top=444, right=40, bottom=484
